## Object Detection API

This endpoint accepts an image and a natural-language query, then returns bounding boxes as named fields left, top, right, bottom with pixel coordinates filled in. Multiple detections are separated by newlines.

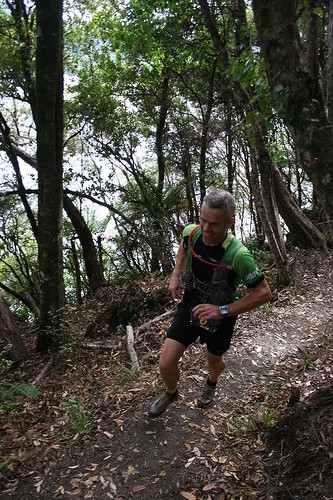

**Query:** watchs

left=219, top=305, right=230, bottom=319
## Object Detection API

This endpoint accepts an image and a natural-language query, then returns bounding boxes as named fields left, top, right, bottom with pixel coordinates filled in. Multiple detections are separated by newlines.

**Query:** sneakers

left=148, top=389, right=179, bottom=417
left=197, top=382, right=216, bottom=409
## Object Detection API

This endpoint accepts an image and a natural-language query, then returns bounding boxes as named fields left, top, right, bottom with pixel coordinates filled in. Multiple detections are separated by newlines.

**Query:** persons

left=148, top=189, right=272, bottom=420
